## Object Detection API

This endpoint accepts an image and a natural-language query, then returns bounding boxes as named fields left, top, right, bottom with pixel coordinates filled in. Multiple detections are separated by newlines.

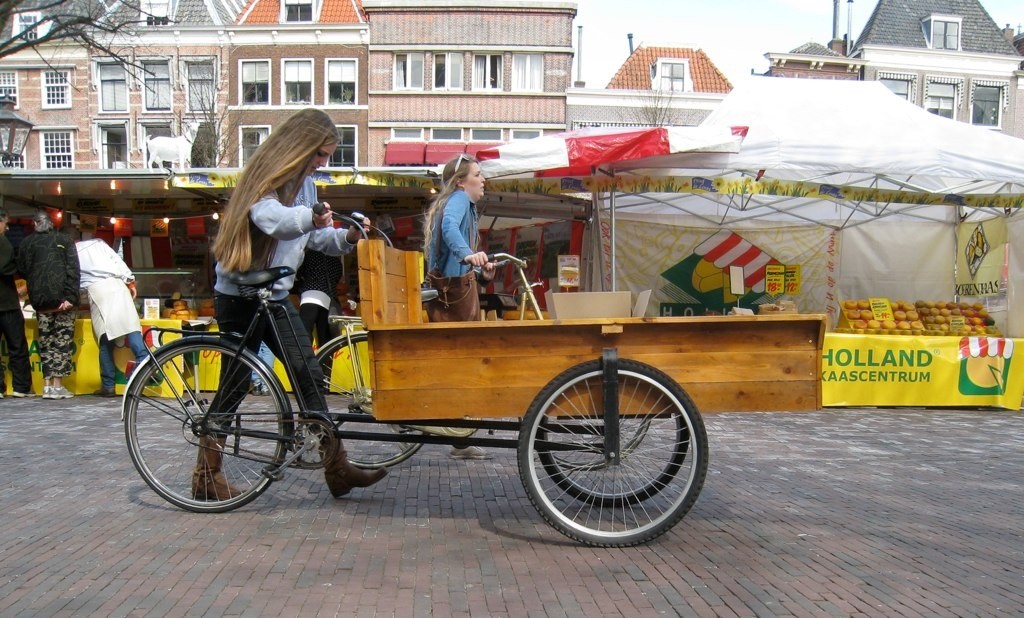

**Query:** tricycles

left=121, top=207, right=827, bottom=549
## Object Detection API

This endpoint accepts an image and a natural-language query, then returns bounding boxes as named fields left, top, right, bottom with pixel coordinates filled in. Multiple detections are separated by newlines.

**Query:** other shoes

left=149, top=371, right=161, bottom=382
left=94, top=388, right=115, bottom=396
left=12, top=389, right=36, bottom=397
left=0, top=393, right=4, bottom=398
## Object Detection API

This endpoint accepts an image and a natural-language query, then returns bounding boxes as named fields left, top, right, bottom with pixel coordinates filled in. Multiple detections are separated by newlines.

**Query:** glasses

left=455, top=154, right=469, bottom=173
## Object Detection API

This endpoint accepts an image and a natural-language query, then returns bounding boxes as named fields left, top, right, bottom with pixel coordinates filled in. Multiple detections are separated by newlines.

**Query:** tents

left=586, top=74, right=1023, bottom=341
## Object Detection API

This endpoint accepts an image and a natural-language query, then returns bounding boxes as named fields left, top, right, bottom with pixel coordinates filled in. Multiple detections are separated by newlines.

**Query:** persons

left=295, top=248, right=344, bottom=394
left=421, top=156, right=497, bottom=321
left=0, top=206, right=37, bottom=399
left=18, top=211, right=81, bottom=400
left=58, top=226, right=161, bottom=397
left=191, top=108, right=389, bottom=500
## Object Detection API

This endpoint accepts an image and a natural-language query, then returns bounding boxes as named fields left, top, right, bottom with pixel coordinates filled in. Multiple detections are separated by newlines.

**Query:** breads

left=845, top=299, right=996, bottom=336
left=162, top=300, right=215, bottom=319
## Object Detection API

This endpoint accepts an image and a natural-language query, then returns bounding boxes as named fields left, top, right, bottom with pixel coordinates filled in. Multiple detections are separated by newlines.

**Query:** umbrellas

left=459, top=124, right=750, bottom=291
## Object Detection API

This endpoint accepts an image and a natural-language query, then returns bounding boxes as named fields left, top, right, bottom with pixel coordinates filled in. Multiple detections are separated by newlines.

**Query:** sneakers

left=448, top=446, right=493, bottom=458
left=51, top=387, right=74, bottom=399
left=42, top=386, right=53, bottom=398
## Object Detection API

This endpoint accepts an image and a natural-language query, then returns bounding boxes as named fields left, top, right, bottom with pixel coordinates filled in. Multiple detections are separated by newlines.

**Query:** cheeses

left=480, top=310, right=497, bottom=321
left=172, top=292, right=182, bottom=300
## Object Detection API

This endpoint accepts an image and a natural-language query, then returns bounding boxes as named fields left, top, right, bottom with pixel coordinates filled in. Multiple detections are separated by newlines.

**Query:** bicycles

left=310, top=248, right=653, bottom=470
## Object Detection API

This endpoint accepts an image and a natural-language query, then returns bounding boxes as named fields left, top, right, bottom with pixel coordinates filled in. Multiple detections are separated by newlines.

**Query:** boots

left=192, top=436, right=246, bottom=500
left=318, top=438, right=389, bottom=497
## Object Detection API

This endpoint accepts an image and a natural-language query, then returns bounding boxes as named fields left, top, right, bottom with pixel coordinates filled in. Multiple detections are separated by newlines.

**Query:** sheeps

left=146, top=122, right=200, bottom=168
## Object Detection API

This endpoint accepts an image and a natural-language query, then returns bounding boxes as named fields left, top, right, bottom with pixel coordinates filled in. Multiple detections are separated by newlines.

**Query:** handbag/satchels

left=422, top=270, right=481, bottom=322
left=26, top=231, right=67, bottom=310
left=328, top=299, right=341, bottom=325
